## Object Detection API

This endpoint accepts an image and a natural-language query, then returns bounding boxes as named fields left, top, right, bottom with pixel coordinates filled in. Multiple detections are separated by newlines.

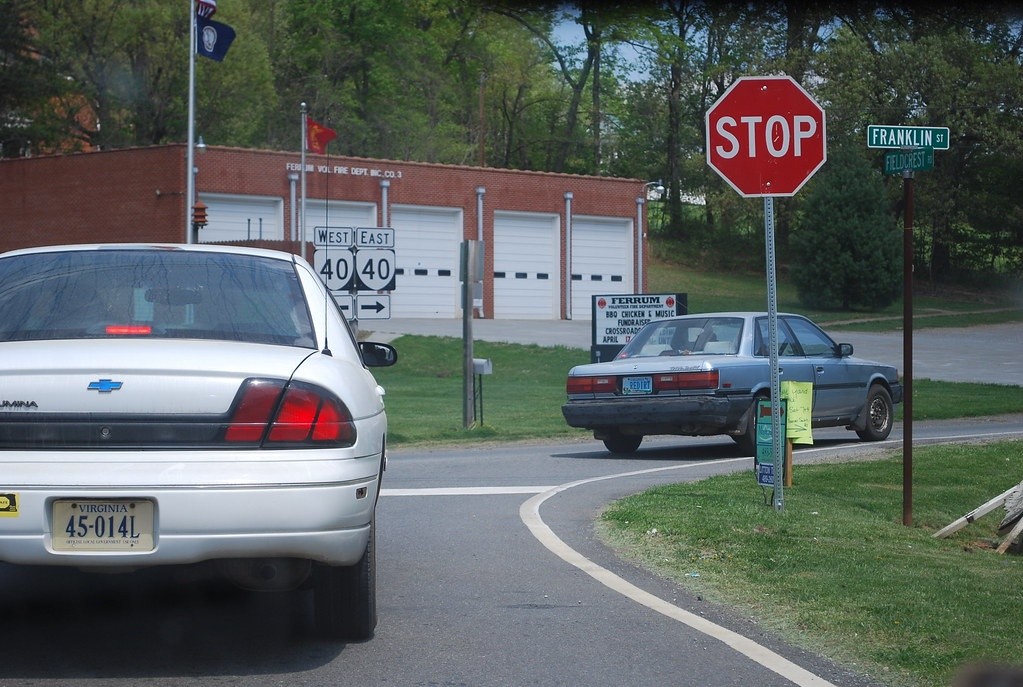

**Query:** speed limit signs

left=314, top=225, right=354, bottom=291
left=355, top=227, right=397, bottom=291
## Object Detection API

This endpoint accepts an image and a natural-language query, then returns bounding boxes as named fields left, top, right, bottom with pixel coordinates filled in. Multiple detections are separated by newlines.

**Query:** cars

left=0, top=239, right=402, bottom=648
left=560, top=310, right=906, bottom=454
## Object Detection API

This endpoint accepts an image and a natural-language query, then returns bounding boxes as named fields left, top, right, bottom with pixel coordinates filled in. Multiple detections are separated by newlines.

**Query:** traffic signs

left=867, top=124, right=949, bottom=151
left=882, top=146, right=935, bottom=176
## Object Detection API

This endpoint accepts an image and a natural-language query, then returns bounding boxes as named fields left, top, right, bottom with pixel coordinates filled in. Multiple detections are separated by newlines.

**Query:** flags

left=195, top=13, right=236, bottom=63
left=304, top=115, right=339, bottom=154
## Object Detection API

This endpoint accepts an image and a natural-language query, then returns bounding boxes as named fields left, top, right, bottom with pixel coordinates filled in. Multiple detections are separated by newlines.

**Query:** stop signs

left=706, top=77, right=827, bottom=200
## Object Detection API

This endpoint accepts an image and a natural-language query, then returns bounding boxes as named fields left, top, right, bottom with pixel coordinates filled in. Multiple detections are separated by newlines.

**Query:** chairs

left=659, top=324, right=691, bottom=357
left=737, top=320, right=768, bottom=356
left=693, top=331, right=716, bottom=352
